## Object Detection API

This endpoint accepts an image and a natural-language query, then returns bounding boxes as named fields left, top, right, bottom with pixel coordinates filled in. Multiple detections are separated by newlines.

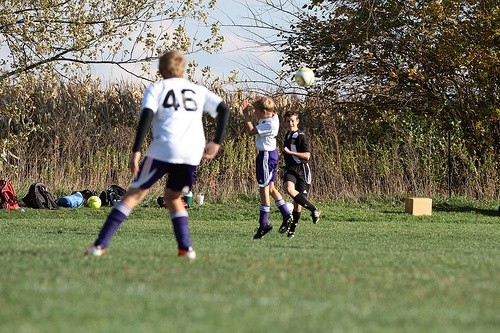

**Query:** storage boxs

left=405, top=197, right=432, bottom=216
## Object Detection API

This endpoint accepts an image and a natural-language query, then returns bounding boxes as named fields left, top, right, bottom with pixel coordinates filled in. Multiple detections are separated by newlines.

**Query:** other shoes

left=178, top=249, right=196, bottom=260
left=83, top=245, right=106, bottom=257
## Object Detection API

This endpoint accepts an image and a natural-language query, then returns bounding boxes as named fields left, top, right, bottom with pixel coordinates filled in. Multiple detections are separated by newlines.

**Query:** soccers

left=87, top=196, right=102, bottom=209
left=286, top=202, right=294, bottom=215
left=294, top=68, right=315, bottom=86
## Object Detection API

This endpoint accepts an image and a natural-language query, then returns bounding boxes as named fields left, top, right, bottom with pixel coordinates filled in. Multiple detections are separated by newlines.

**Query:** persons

left=85, top=51, right=229, bottom=260
left=241, top=96, right=293, bottom=238
left=282, top=111, right=320, bottom=237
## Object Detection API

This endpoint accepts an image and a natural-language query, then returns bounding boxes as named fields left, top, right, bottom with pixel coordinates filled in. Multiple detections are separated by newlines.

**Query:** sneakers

left=310, top=206, right=321, bottom=224
left=279, top=216, right=292, bottom=233
left=254, top=225, right=273, bottom=239
left=288, top=223, right=297, bottom=237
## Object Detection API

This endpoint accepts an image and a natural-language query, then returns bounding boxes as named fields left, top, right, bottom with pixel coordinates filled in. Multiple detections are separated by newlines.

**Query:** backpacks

left=22, top=182, right=60, bottom=211
left=57, top=184, right=129, bottom=209
left=0, top=180, right=20, bottom=211
